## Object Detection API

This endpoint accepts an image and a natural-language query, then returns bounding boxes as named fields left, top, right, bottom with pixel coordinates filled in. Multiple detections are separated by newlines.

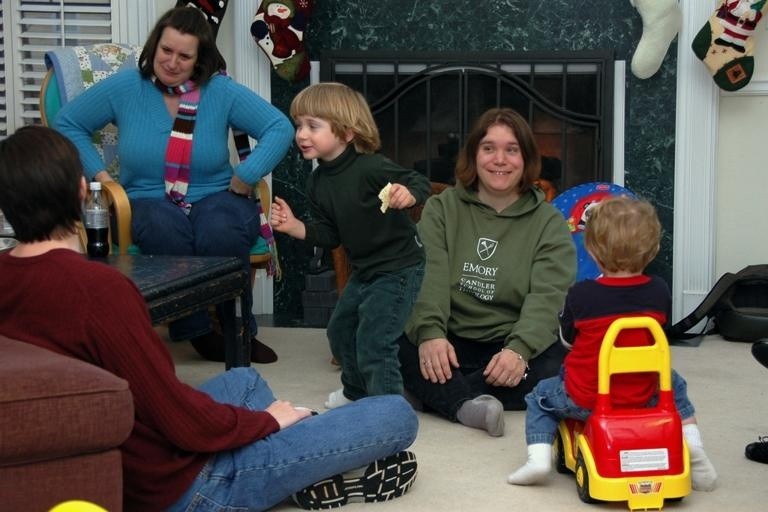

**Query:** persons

left=52, top=5, right=295, bottom=365
left=402, top=102, right=575, bottom=434
left=508, top=193, right=718, bottom=491
left=0, top=124, right=419, bottom=509
left=265, top=80, right=433, bottom=411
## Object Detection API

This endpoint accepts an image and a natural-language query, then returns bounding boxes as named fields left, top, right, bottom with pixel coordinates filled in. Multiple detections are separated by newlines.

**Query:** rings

left=422, top=360, right=431, bottom=366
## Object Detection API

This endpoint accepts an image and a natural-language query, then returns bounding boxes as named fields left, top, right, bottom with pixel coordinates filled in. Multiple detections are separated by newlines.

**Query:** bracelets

left=501, top=348, right=531, bottom=370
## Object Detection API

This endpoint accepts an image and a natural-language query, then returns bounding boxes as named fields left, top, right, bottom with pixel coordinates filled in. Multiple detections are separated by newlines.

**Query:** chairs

left=39, top=41, right=273, bottom=336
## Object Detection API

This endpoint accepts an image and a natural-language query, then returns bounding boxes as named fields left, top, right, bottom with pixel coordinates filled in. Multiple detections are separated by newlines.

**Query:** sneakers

left=290, top=449, right=419, bottom=510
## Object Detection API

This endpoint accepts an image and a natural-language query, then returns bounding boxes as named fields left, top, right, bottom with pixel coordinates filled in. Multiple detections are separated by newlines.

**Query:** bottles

left=82, top=181, right=110, bottom=261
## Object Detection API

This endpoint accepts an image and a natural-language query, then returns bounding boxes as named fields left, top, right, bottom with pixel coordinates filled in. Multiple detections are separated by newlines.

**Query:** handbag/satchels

left=712, top=263, right=768, bottom=343
